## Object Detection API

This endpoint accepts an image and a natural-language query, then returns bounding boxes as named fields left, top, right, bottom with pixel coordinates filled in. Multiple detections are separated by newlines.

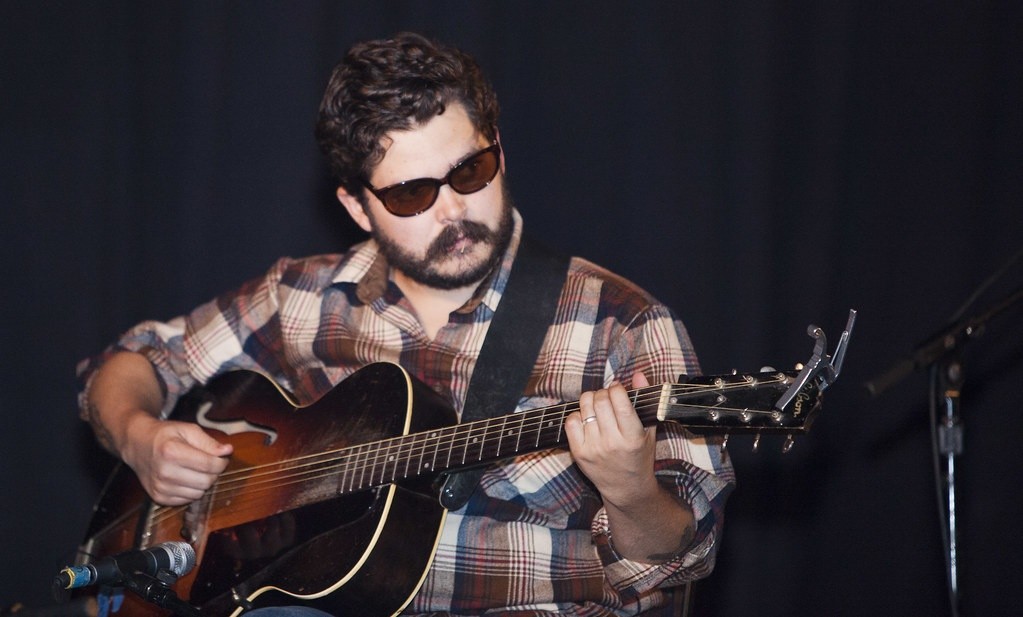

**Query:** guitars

left=74, top=307, right=857, bottom=617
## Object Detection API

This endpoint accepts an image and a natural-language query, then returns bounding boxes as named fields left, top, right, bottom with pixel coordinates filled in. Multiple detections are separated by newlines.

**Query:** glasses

left=360, top=119, right=500, bottom=217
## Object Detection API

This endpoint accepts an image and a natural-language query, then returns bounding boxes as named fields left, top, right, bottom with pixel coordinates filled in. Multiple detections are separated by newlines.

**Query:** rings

left=583, top=416, right=597, bottom=424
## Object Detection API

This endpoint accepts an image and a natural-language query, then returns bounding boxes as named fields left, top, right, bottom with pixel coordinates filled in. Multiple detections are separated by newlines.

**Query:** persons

left=74, top=31, right=733, bottom=616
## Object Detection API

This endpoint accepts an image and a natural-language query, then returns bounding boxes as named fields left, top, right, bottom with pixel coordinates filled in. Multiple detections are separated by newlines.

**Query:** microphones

left=50, top=541, right=196, bottom=592
